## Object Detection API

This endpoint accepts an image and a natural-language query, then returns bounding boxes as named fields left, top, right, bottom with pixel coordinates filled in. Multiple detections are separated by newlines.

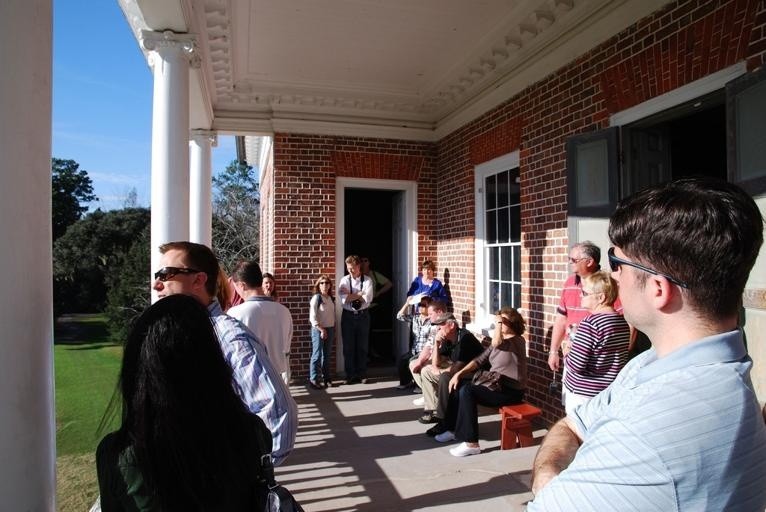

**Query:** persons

left=548, top=241, right=637, bottom=373
left=94, top=292, right=276, bottom=512
left=561, top=272, right=631, bottom=416
left=148, top=237, right=300, bottom=473
left=524, top=173, right=765, bottom=512
left=396, top=259, right=490, bottom=437
left=309, top=274, right=334, bottom=389
left=338, top=256, right=391, bottom=384
left=215, top=262, right=293, bottom=390
left=435, top=307, right=524, bottom=456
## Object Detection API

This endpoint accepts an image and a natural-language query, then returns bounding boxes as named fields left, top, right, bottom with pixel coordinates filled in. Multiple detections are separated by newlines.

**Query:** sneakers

left=397, top=381, right=481, bottom=457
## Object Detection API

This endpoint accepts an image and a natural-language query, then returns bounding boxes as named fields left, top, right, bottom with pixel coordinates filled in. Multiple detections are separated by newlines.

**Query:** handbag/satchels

left=472, top=369, right=501, bottom=391
left=265, top=486, right=305, bottom=512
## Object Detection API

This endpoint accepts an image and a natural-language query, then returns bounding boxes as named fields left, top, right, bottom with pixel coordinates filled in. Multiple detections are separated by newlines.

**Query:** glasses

left=568, top=257, right=590, bottom=263
left=608, top=247, right=690, bottom=289
left=155, top=267, right=197, bottom=282
left=582, top=290, right=601, bottom=296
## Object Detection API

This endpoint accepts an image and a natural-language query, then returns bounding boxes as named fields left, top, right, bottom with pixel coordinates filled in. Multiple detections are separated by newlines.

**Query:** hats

left=431, top=312, right=456, bottom=325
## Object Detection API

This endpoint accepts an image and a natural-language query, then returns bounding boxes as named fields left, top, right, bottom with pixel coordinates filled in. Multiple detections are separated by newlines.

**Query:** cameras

left=550, top=382, right=558, bottom=395
left=351, top=299, right=362, bottom=310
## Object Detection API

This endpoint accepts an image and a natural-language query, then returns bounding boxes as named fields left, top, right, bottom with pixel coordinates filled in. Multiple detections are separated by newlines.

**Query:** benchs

left=496, top=402, right=542, bottom=451
